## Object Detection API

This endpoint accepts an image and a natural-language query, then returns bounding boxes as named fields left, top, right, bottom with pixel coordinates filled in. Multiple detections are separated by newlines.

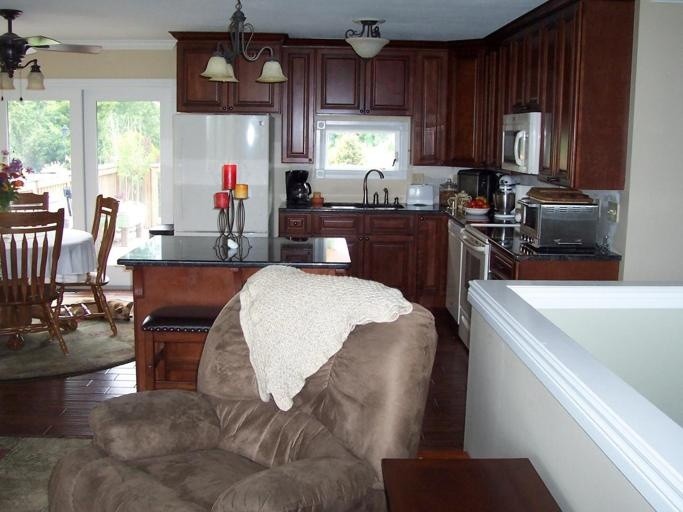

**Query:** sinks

left=332, top=206, right=397, bottom=213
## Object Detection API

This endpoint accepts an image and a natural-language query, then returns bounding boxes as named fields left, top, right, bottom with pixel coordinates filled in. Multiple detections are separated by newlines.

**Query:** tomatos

left=467, top=200, right=489, bottom=208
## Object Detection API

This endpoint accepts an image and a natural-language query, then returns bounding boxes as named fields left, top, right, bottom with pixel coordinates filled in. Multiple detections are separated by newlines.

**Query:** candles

left=234, top=183, right=248, bottom=199
left=212, top=191, right=229, bottom=209
left=221, top=164, right=237, bottom=190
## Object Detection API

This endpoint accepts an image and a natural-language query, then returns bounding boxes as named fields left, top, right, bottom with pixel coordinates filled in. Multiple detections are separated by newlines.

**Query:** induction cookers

left=465, top=224, right=520, bottom=244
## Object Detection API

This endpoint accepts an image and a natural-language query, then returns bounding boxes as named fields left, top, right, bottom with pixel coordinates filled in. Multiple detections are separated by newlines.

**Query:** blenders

left=489, top=173, right=519, bottom=219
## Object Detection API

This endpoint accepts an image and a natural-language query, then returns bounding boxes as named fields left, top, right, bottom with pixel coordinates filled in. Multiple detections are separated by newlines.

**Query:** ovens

left=456, top=231, right=491, bottom=352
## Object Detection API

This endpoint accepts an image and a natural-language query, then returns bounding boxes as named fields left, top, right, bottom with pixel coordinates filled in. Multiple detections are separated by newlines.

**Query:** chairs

left=0, top=207, right=68, bottom=352
left=43, top=261, right=440, bottom=511
left=5, top=191, right=51, bottom=224
left=49, top=195, right=123, bottom=341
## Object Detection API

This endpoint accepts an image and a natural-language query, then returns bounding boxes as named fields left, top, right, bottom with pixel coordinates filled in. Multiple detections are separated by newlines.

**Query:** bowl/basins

left=463, top=208, right=489, bottom=215
left=310, top=198, right=323, bottom=209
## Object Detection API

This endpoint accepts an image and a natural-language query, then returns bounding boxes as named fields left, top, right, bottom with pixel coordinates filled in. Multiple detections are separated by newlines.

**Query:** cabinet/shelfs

left=315, top=40, right=411, bottom=117
left=412, top=42, right=476, bottom=166
left=541, top=1, right=633, bottom=191
left=278, top=210, right=316, bottom=237
left=316, top=210, right=416, bottom=302
left=175, top=34, right=282, bottom=113
left=487, top=238, right=622, bottom=281
left=478, top=23, right=512, bottom=167
left=282, top=37, right=315, bottom=165
left=417, top=209, right=446, bottom=307
left=510, top=7, right=544, bottom=113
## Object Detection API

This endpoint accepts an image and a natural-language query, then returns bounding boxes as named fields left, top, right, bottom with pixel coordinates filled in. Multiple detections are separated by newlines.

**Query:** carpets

left=0, top=433, right=92, bottom=512
left=0, top=316, right=135, bottom=384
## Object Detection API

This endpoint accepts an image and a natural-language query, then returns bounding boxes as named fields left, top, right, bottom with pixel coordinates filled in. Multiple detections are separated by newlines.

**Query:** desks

left=1, top=225, right=98, bottom=336
left=378, top=456, right=563, bottom=511
left=116, top=233, right=354, bottom=393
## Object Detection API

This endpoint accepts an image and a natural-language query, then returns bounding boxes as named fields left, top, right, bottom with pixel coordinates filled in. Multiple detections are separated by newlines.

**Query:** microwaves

left=517, top=197, right=600, bottom=253
left=456, top=169, right=503, bottom=208
left=498, top=113, right=542, bottom=176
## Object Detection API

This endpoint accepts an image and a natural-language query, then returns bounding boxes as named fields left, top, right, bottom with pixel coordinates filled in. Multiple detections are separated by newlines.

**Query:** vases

left=0, top=196, right=12, bottom=228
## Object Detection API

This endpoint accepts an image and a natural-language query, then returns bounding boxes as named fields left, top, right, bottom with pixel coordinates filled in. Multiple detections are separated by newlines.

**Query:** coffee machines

left=284, top=168, right=311, bottom=208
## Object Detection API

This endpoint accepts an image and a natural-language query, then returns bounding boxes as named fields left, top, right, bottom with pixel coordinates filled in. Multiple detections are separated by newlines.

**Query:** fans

left=0, top=8, right=103, bottom=57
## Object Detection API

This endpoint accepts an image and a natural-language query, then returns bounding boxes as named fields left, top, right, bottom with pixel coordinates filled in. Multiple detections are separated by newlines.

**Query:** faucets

left=382, top=188, right=389, bottom=202
left=363, top=168, right=384, bottom=204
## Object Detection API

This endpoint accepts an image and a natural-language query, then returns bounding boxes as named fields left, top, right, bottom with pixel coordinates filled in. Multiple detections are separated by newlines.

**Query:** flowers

left=0, top=151, right=32, bottom=207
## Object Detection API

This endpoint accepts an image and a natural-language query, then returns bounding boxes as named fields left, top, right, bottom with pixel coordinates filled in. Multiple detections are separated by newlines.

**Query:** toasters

left=405, top=183, right=435, bottom=207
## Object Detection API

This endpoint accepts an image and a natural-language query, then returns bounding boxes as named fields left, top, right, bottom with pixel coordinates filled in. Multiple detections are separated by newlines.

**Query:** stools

left=141, top=303, right=226, bottom=393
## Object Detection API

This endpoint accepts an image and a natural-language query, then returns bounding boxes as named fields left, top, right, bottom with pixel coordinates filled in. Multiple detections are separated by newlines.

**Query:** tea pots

left=454, top=190, right=471, bottom=213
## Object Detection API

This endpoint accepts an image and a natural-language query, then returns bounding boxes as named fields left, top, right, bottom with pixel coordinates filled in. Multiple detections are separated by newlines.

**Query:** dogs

left=68, top=299, right=133, bottom=322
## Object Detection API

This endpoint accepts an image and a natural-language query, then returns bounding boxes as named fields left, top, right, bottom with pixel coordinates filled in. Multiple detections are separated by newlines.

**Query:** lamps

left=200, top=1, right=289, bottom=85
left=343, top=14, right=391, bottom=59
left=0, top=59, right=47, bottom=91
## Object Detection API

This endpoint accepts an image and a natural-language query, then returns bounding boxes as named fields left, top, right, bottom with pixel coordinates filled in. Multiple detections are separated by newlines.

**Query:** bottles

left=438, top=178, right=457, bottom=210
